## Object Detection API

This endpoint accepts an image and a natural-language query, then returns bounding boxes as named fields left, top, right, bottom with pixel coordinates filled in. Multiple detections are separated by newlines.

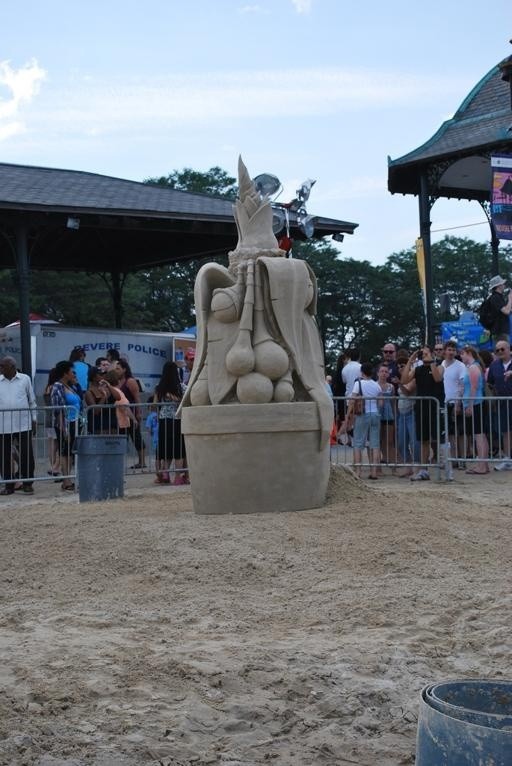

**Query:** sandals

left=45, top=470, right=75, bottom=493
left=129, top=463, right=148, bottom=469
left=348, top=457, right=413, bottom=480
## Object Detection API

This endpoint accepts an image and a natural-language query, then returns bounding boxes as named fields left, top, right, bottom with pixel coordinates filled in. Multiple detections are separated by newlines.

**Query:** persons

left=323, top=339, right=512, bottom=482
left=477, top=274, right=511, bottom=346
left=42, top=343, right=197, bottom=495
left=0, top=353, right=38, bottom=497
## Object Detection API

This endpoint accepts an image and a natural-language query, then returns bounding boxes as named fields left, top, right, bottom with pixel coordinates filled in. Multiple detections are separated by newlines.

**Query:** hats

left=184, top=347, right=196, bottom=360
left=487, top=275, right=507, bottom=291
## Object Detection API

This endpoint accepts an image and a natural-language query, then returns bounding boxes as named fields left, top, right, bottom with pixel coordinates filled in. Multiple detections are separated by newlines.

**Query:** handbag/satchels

left=337, top=423, right=353, bottom=447
left=352, top=392, right=365, bottom=416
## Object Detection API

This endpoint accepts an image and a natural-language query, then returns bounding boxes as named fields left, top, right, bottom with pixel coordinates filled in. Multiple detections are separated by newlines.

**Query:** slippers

left=464, top=464, right=490, bottom=475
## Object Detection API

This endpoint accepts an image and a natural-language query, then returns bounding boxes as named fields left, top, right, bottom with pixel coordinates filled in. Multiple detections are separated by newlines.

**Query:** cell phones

left=418, top=351, right=423, bottom=360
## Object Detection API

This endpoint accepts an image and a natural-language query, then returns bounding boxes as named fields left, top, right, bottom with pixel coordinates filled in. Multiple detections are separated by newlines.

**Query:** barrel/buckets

left=70, top=434, right=128, bottom=504
left=412, top=678, right=512, bottom=766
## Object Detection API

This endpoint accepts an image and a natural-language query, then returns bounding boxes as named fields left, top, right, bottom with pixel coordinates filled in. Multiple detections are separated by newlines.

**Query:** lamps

left=251, top=171, right=320, bottom=240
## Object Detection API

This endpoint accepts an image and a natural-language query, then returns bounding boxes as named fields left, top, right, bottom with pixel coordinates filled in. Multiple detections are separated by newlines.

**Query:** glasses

left=495, top=347, right=505, bottom=353
left=396, top=365, right=405, bottom=370
left=434, top=348, right=444, bottom=351
left=384, top=349, right=396, bottom=355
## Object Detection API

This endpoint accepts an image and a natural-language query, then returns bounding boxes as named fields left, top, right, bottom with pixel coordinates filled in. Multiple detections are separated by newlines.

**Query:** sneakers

left=493, top=458, right=512, bottom=472
left=15, top=485, right=34, bottom=496
left=409, top=470, right=431, bottom=481
left=0, top=488, right=16, bottom=496
left=444, top=469, right=455, bottom=481
left=154, top=470, right=191, bottom=486
left=452, top=456, right=469, bottom=471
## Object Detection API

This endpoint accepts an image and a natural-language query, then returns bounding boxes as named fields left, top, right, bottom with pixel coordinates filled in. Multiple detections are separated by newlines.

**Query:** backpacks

left=479, top=294, right=499, bottom=331
left=483, top=382, right=498, bottom=414
